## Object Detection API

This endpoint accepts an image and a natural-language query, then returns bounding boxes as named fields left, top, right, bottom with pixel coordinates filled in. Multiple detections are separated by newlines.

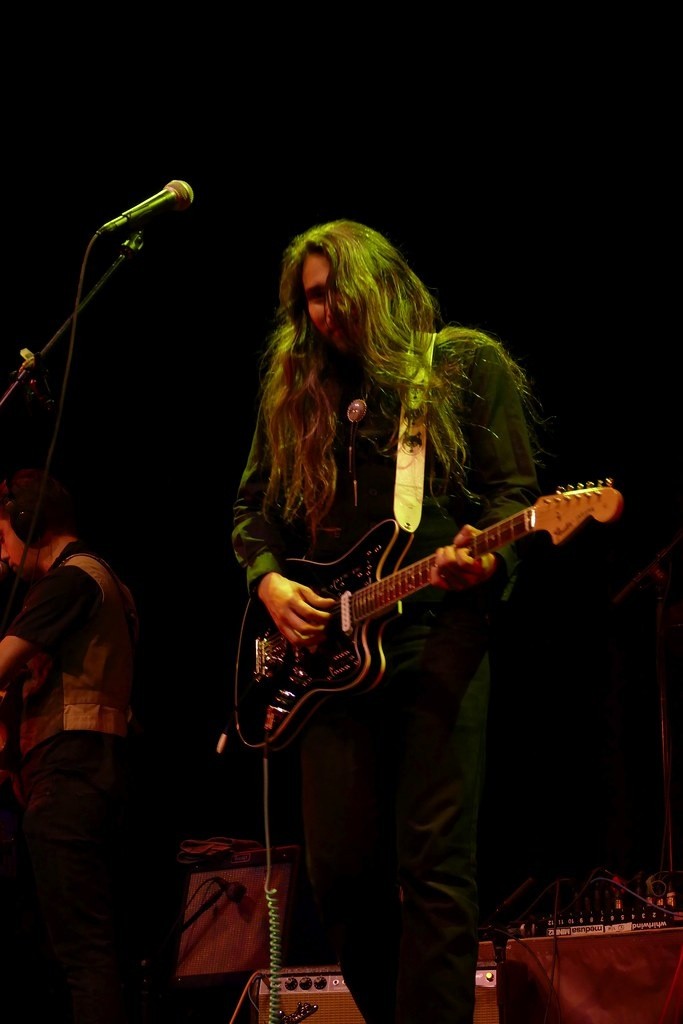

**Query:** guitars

left=232, top=478, right=622, bottom=750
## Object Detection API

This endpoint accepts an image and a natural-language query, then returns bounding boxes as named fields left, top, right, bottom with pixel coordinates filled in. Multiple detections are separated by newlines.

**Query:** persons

left=0, top=469, right=137, bottom=1024
left=228, top=221, right=535, bottom=1023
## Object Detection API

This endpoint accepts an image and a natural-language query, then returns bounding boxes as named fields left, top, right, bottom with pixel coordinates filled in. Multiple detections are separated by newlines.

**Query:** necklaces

left=344, top=367, right=371, bottom=511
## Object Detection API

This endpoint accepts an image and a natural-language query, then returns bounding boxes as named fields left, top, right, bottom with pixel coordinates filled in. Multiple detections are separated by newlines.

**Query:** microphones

left=97, top=180, right=194, bottom=233
